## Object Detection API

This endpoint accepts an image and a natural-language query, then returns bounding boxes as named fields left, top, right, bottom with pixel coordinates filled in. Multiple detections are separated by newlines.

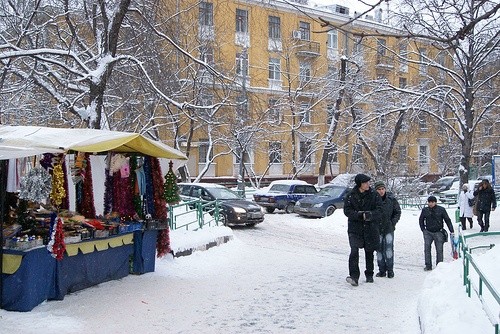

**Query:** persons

left=344, top=173, right=382, bottom=286
left=419, top=196, right=454, bottom=270
left=457, top=184, right=474, bottom=230
left=374, top=180, right=401, bottom=278
left=473, top=179, right=496, bottom=233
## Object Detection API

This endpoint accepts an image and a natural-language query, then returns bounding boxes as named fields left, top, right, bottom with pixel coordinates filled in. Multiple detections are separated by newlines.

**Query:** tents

left=0, top=124, right=188, bottom=312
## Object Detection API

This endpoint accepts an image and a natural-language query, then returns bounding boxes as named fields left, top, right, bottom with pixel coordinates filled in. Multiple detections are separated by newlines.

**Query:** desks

left=0, top=228, right=158, bottom=312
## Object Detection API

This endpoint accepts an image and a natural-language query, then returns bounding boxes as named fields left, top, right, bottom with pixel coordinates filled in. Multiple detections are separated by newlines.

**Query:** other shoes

left=480, top=227, right=484, bottom=232
left=462, top=227, right=466, bottom=230
left=470, top=222, right=473, bottom=228
left=346, top=277, right=358, bottom=286
left=376, top=272, right=386, bottom=277
left=484, top=228, right=488, bottom=231
left=388, top=271, right=394, bottom=278
left=367, top=275, right=374, bottom=282
left=424, top=266, right=432, bottom=271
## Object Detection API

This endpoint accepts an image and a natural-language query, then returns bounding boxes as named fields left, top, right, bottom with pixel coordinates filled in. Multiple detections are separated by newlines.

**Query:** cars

left=293, top=185, right=353, bottom=218
left=387, top=175, right=492, bottom=204
left=252, top=173, right=376, bottom=214
left=177, top=182, right=264, bottom=228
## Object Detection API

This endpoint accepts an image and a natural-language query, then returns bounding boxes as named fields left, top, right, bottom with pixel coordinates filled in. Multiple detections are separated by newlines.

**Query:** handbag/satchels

left=468, top=190, right=477, bottom=207
left=443, top=229, right=448, bottom=242
left=455, top=208, right=460, bottom=223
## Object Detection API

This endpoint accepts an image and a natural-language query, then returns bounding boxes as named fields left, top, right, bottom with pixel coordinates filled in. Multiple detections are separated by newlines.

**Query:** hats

left=481, top=179, right=490, bottom=186
left=374, top=180, right=386, bottom=190
left=462, top=183, right=470, bottom=189
left=355, top=174, right=373, bottom=185
left=428, top=196, right=437, bottom=202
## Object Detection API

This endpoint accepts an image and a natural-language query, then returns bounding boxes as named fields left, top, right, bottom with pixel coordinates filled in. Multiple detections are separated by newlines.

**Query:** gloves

left=358, top=211, right=372, bottom=221
